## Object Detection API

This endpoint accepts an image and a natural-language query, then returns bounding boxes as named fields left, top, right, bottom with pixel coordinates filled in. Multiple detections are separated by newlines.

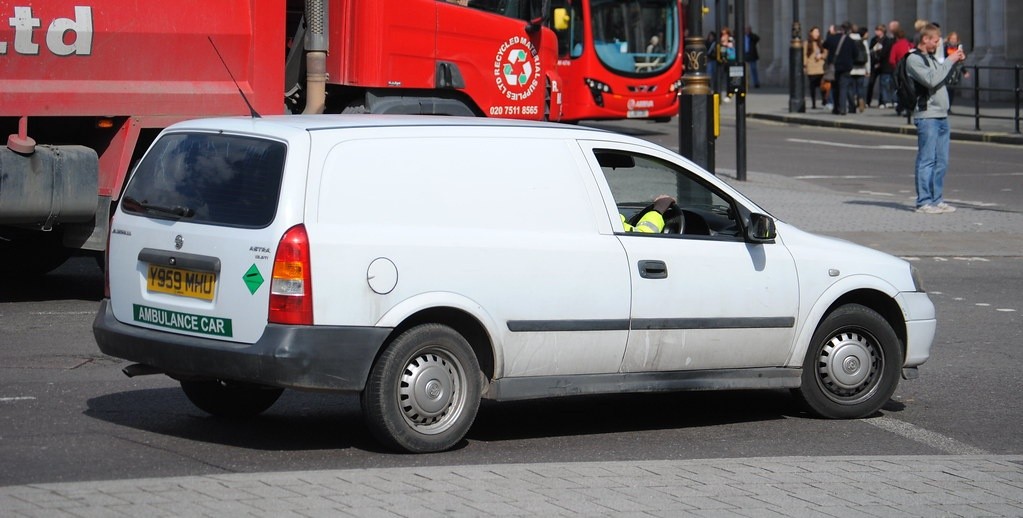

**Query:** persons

left=905, top=23, right=966, bottom=214
left=683, top=25, right=760, bottom=102
left=803, top=19, right=970, bottom=124
left=621, top=194, right=675, bottom=235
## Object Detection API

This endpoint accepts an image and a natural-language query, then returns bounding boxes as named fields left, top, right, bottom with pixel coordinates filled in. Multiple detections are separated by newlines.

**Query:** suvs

left=91, top=111, right=937, bottom=454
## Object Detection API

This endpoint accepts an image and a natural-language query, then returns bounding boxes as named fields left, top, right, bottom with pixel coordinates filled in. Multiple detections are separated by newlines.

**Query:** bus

left=541, top=0, right=686, bottom=125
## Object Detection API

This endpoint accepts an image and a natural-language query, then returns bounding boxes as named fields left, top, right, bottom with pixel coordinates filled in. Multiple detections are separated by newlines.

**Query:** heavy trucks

left=0, top=0, right=561, bottom=284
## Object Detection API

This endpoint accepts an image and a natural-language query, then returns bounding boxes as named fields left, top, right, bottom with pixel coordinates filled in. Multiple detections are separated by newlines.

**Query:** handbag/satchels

left=822, top=62, right=835, bottom=81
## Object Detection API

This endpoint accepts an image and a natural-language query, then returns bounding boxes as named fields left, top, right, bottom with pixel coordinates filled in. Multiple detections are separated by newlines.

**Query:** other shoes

left=857, top=97, right=865, bottom=114
left=915, top=202, right=956, bottom=214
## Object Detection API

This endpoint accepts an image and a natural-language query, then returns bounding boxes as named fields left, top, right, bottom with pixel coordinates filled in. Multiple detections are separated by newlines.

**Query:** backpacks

left=894, top=51, right=936, bottom=124
